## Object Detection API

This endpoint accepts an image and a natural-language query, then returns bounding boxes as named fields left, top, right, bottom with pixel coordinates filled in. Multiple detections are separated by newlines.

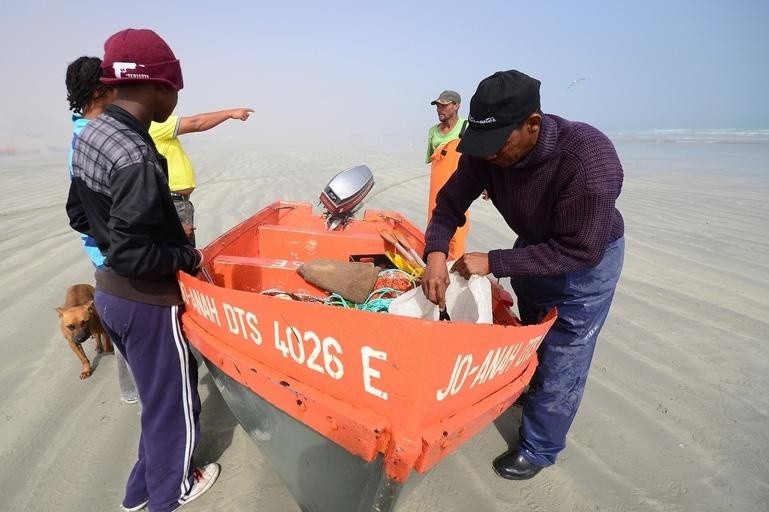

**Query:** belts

left=172, top=195, right=190, bottom=200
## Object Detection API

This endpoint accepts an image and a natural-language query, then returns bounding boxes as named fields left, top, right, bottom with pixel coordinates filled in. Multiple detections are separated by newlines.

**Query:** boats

left=181, top=166, right=562, bottom=511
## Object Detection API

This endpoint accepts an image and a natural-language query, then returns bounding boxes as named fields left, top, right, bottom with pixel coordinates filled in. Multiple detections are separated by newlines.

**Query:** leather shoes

left=492, top=450, right=542, bottom=480
left=513, top=386, right=530, bottom=405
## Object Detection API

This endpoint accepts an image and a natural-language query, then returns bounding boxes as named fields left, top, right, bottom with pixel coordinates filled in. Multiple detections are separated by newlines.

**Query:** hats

left=99, top=29, right=183, bottom=91
left=431, top=91, right=461, bottom=106
left=455, top=70, right=541, bottom=157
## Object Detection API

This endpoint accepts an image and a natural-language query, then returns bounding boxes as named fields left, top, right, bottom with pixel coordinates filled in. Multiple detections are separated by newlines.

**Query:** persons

left=426, top=91, right=489, bottom=262
left=148, top=108, right=256, bottom=248
left=66, top=56, right=142, bottom=416
left=421, top=69, right=625, bottom=480
left=65, top=28, right=221, bottom=512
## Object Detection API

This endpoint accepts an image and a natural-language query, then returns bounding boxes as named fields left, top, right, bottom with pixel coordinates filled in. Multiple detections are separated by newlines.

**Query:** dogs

left=53, top=284, right=114, bottom=380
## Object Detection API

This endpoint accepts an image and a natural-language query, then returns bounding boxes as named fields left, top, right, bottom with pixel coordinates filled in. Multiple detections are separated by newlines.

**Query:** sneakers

left=171, top=462, right=220, bottom=511
left=118, top=498, right=148, bottom=512
